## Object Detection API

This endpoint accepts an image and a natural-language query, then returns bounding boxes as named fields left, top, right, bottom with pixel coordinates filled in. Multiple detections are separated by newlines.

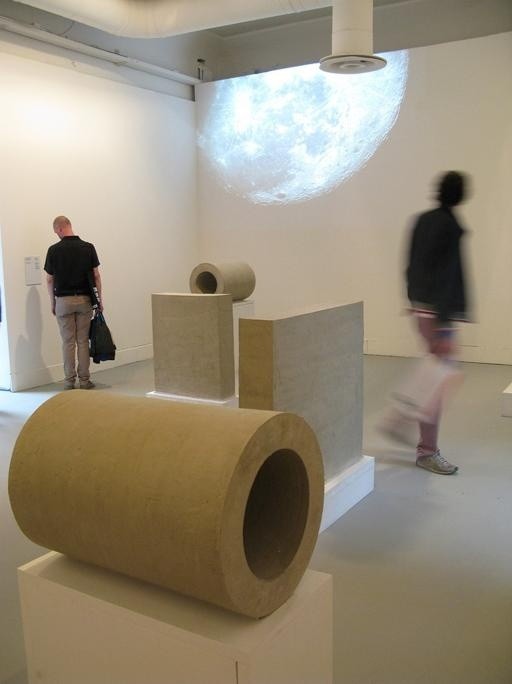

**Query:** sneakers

left=80, top=382, right=94, bottom=389
left=416, top=451, right=458, bottom=475
left=64, top=384, right=74, bottom=389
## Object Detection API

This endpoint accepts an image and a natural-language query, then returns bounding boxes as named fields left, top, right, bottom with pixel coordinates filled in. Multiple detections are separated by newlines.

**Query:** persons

left=374, top=171, right=476, bottom=474
left=43, top=216, right=103, bottom=390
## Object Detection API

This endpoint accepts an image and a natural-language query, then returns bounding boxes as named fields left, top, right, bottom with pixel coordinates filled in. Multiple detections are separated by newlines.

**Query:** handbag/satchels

left=89, top=319, right=116, bottom=363
